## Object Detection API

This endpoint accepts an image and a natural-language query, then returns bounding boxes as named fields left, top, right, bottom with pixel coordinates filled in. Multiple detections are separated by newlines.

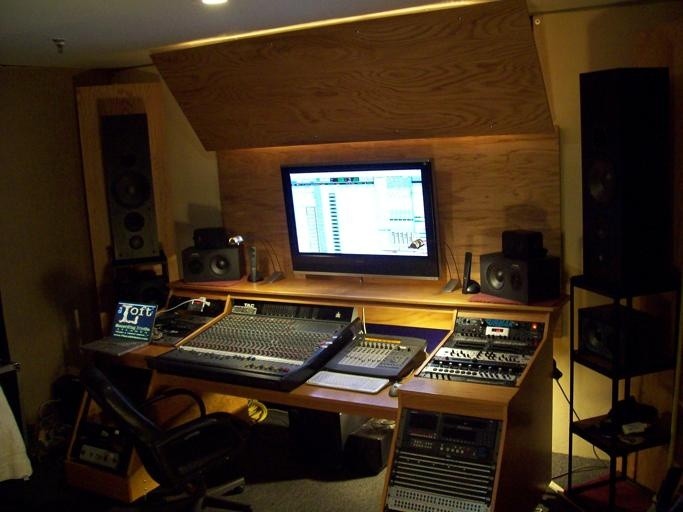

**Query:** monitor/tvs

left=280, top=158, right=442, bottom=281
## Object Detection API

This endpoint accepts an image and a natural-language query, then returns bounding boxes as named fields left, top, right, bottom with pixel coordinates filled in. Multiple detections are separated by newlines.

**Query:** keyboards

left=305, top=370, right=389, bottom=393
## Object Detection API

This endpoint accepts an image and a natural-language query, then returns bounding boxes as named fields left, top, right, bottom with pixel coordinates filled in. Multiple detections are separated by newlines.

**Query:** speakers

left=578, top=303, right=665, bottom=367
left=480, top=251, right=559, bottom=304
left=193, top=228, right=227, bottom=249
left=578, top=64, right=673, bottom=292
left=182, top=247, right=244, bottom=283
left=501, top=229, right=544, bottom=259
left=98, top=113, right=160, bottom=260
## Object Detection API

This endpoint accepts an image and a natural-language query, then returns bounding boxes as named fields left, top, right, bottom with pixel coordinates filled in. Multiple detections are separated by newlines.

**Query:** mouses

left=389, top=382, right=403, bottom=397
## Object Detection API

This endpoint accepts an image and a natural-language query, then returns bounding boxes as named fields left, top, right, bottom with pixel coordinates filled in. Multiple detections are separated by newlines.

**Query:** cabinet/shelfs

left=566, top=271, right=681, bottom=512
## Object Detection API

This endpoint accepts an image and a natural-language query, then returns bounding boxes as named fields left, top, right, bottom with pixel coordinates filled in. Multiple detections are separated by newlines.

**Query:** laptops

left=80, top=300, right=159, bottom=357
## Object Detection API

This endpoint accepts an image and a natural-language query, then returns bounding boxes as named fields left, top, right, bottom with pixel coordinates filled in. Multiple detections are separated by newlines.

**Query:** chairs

left=79, top=366, right=254, bottom=511
